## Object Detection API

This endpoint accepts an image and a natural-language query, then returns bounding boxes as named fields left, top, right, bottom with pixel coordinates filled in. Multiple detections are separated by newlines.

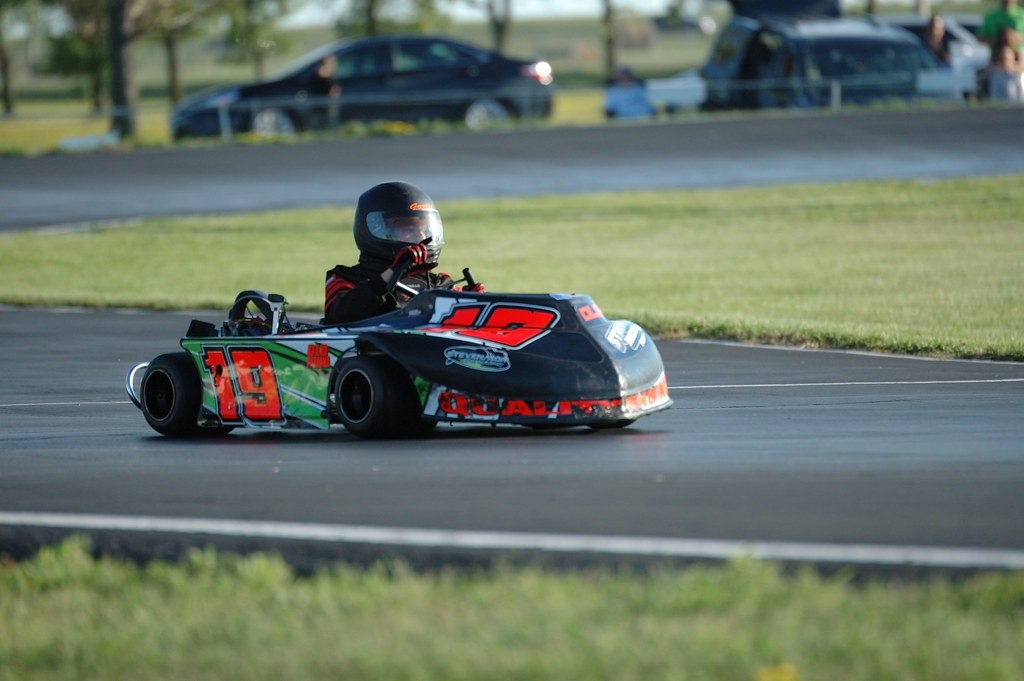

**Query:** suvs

left=703, top=9, right=965, bottom=110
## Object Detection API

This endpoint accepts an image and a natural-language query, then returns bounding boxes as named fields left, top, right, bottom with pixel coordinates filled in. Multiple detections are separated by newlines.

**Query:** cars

left=170, top=32, right=553, bottom=140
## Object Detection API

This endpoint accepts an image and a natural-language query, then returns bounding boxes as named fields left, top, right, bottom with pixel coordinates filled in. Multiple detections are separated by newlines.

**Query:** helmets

left=353, top=182, right=445, bottom=270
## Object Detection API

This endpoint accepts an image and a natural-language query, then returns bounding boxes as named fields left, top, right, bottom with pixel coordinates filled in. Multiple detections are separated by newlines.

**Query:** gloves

left=390, top=236, right=438, bottom=280
left=462, top=283, right=485, bottom=293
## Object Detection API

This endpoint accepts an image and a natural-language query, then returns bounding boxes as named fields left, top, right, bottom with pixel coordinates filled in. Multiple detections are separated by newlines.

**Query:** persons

left=324, top=183, right=485, bottom=326
left=977, top=0, right=1024, bottom=72
left=298, top=56, right=342, bottom=129
left=924, top=13, right=955, bottom=68
